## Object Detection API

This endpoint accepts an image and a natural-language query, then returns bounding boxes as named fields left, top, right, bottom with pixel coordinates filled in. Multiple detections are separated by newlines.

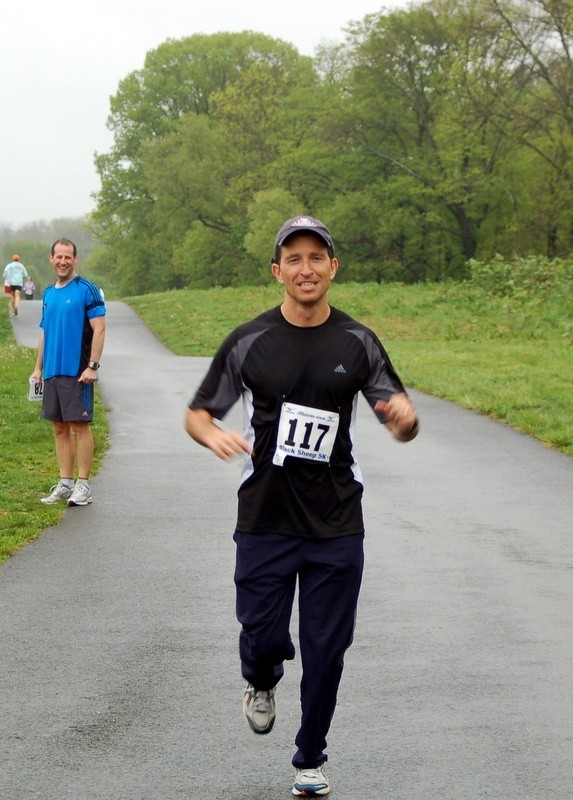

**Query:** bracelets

left=411, top=417, right=419, bottom=433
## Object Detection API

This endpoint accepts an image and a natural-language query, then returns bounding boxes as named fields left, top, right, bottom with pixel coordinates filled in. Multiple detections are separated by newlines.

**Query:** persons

left=185, top=216, right=421, bottom=798
left=28, top=238, right=105, bottom=506
left=3, top=255, right=28, bottom=316
left=23, top=275, right=35, bottom=301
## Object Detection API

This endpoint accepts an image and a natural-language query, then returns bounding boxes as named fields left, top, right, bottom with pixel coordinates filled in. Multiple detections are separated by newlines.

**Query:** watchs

left=88, top=361, right=101, bottom=370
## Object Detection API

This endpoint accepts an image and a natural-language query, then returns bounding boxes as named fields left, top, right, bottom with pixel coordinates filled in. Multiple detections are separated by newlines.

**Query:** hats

left=271, top=217, right=335, bottom=258
left=12, top=255, right=20, bottom=260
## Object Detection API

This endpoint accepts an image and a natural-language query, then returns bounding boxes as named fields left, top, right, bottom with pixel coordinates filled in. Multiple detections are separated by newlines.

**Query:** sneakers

left=292, top=764, right=331, bottom=797
left=67, top=479, right=94, bottom=505
left=39, top=476, right=74, bottom=505
left=241, top=682, right=278, bottom=734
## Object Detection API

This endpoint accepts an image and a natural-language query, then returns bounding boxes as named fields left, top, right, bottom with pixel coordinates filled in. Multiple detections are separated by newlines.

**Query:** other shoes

left=12, top=307, right=18, bottom=316
